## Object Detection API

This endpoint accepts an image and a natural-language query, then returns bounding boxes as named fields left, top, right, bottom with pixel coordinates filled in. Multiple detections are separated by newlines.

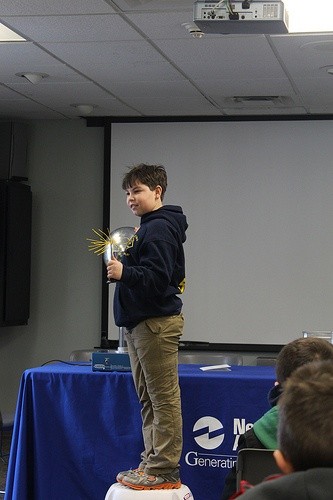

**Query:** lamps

left=76, top=106, right=94, bottom=115
left=23, top=74, right=42, bottom=85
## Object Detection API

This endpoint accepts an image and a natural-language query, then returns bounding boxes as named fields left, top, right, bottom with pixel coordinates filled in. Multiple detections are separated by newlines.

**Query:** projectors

left=194, top=0, right=288, bottom=35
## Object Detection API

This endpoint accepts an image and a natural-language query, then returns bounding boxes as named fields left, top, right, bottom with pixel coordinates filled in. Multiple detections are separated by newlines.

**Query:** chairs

left=237, top=448, right=282, bottom=494
left=177, top=355, right=243, bottom=366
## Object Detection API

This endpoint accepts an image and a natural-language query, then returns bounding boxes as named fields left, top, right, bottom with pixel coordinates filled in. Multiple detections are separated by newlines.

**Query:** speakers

left=0, top=181, right=32, bottom=325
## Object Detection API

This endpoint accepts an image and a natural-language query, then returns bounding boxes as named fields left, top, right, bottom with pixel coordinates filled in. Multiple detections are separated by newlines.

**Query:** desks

left=5, top=360, right=280, bottom=500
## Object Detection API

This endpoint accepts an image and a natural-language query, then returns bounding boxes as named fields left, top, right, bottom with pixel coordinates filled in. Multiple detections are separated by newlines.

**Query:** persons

left=106, top=165, right=189, bottom=490
left=221, top=336, right=333, bottom=500
left=236, top=360, right=333, bottom=500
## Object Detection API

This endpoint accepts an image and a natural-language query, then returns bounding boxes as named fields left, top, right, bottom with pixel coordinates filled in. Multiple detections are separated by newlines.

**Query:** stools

left=105, top=483, right=195, bottom=500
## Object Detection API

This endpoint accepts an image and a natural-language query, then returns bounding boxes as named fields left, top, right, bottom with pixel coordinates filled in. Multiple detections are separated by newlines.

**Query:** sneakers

left=116, top=466, right=181, bottom=490
